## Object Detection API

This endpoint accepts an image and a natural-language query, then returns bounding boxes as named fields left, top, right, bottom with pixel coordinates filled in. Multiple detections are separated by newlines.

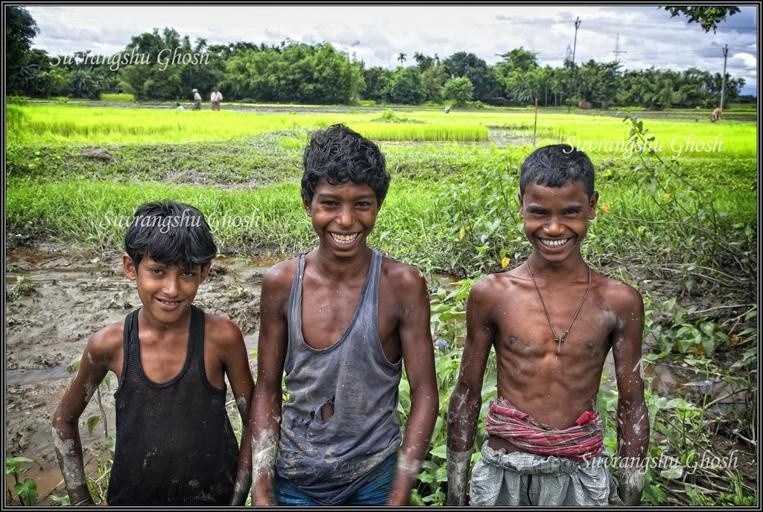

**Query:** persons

left=209, top=87, right=223, bottom=111
left=48, top=197, right=254, bottom=507
left=250, top=122, right=441, bottom=505
left=176, top=102, right=184, bottom=109
left=190, top=88, right=202, bottom=110
left=711, top=104, right=726, bottom=124
left=444, top=144, right=650, bottom=506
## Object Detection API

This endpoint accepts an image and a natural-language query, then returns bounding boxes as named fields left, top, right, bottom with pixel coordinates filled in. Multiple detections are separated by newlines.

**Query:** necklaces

left=524, top=256, right=593, bottom=352
